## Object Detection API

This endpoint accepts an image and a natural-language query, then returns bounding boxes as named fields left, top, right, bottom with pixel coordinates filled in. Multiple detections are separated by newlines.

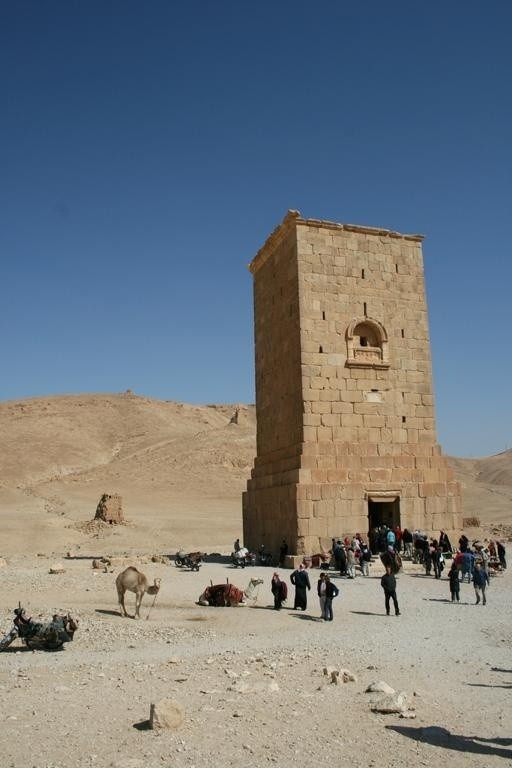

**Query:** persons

left=290, top=563, right=310, bottom=610
left=323, top=574, right=338, bottom=621
left=470, top=563, right=490, bottom=605
left=381, top=565, right=402, bottom=615
left=447, top=564, right=460, bottom=604
left=234, top=539, right=289, bottom=568
left=317, top=523, right=507, bottom=581
left=271, top=571, right=287, bottom=611
left=318, top=573, right=329, bottom=618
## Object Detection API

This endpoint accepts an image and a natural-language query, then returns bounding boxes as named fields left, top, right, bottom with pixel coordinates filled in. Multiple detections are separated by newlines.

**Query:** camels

left=115, top=566, right=162, bottom=620
left=195, top=575, right=264, bottom=608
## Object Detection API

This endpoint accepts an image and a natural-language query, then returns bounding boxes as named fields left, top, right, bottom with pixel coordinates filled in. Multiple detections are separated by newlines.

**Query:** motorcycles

left=231, top=543, right=273, bottom=568
left=1, top=601, right=79, bottom=653
left=175, top=546, right=202, bottom=572
